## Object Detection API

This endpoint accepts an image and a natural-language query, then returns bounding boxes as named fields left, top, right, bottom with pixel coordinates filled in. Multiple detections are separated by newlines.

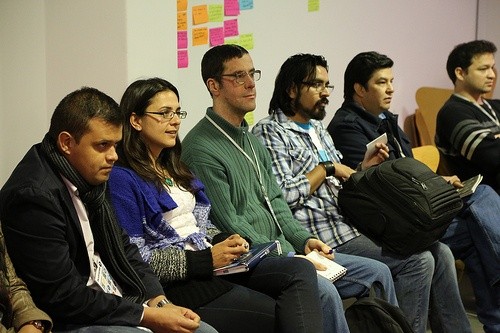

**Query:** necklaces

left=155, top=163, right=173, bottom=186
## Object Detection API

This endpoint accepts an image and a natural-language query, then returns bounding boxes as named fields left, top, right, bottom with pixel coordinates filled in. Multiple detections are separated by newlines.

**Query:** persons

left=434, top=40, right=500, bottom=196
left=326, top=51, right=500, bottom=333
left=0, top=220, right=53, bottom=333
left=107, top=77, right=323, bottom=333
left=252, top=53, right=472, bottom=333
left=180, top=44, right=399, bottom=333
left=0, top=86, right=219, bottom=333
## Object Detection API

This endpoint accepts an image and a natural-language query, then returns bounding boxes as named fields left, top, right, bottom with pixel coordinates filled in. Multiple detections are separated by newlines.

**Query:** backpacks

left=336, top=156, right=464, bottom=260
left=344, top=279, right=414, bottom=333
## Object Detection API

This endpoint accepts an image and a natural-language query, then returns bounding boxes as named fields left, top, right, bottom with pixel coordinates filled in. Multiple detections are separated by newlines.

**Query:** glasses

left=301, top=81, right=334, bottom=93
left=209, top=70, right=261, bottom=85
left=138, top=110, right=188, bottom=119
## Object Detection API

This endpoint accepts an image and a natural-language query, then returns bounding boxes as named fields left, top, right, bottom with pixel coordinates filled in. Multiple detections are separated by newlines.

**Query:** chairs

left=408, top=86, right=465, bottom=285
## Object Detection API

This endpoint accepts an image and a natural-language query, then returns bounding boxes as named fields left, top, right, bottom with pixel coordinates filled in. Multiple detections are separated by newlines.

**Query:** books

left=456, top=174, right=483, bottom=199
left=213, top=242, right=277, bottom=276
left=306, top=251, right=347, bottom=283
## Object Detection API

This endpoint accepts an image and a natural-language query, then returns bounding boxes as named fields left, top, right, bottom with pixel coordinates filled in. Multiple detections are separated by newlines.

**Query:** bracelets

left=154, top=299, right=170, bottom=308
left=318, top=161, right=336, bottom=177
left=28, top=320, right=45, bottom=332
